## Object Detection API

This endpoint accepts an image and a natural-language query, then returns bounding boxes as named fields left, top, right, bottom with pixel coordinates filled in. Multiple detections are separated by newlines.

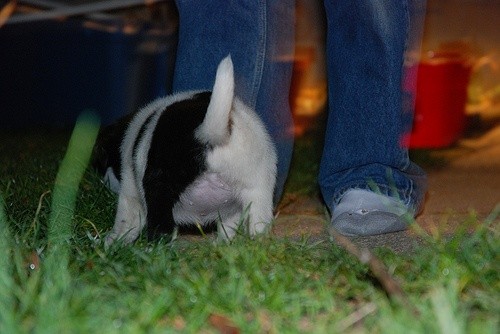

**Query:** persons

left=103, top=0, right=429, bottom=234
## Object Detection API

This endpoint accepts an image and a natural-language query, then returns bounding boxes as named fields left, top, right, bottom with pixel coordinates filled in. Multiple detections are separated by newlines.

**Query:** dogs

left=90, top=52, right=279, bottom=253
